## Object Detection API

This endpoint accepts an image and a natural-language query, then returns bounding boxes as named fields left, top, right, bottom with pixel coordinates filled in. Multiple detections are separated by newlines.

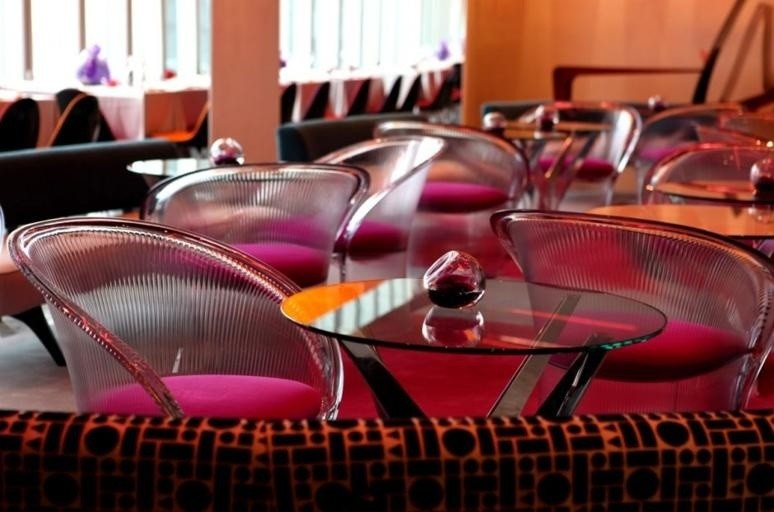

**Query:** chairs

left=0, top=63, right=773, bottom=420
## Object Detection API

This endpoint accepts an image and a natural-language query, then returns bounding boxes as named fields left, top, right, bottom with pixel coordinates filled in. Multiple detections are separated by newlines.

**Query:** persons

left=77, top=45, right=113, bottom=86
left=439, top=39, right=449, bottom=60
